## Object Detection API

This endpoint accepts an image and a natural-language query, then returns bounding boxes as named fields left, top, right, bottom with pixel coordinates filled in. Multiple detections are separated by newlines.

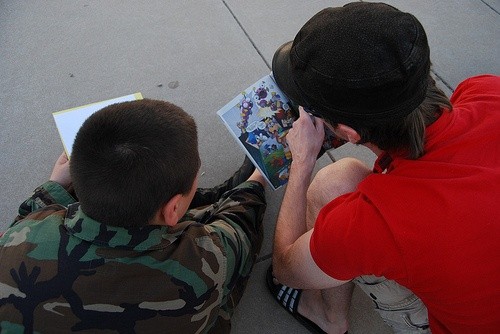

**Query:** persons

left=264, top=2, right=500, bottom=333
left=1, top=97, right=268, bottom=334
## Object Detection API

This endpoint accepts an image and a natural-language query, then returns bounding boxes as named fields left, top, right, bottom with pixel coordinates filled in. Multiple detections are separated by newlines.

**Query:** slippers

left=265, top=263, right=348, bottom=333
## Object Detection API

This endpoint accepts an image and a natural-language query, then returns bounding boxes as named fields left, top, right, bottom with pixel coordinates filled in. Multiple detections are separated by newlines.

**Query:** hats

left=272, top=2, right=431, bottom=125
